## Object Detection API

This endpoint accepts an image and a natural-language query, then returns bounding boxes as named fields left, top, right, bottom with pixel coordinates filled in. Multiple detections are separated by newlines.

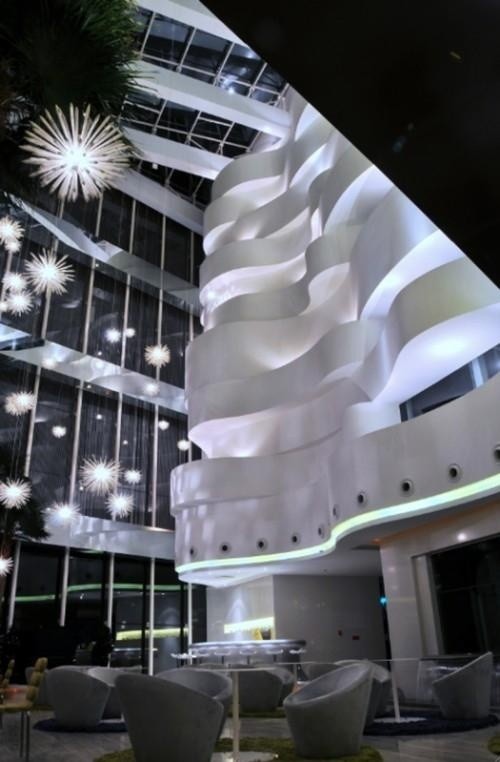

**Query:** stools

left=0, top=702, right=33, bottom=762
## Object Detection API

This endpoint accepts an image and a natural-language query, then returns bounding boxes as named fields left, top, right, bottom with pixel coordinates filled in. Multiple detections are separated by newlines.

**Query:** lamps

left=50, top=425, right=68, bottom=440
left=46, top=497, right=81, bottom=525
left=143, top=379, right=161, bottom=397
left=20, top=101, right=130, bottom=207
left=3, top=389, right=36, bottom=419
left=1, top=475, right=32, bottom=511
left=0, top=207, right=76, bottom=317
left=79, top=452, right=144, bottom=519
left=96, top=323, right=171, bottom=371
left=158, top=420, right=170, bottom=431
left=0, top=552, right=17, bottom=577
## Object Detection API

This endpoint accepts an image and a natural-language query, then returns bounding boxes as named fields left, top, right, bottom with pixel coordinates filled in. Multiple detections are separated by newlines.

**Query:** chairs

left=114, top=672, right=223, bottom=761
left=50, top=663, right=143, bottom=734
left=158, top=666, right=234, bottom=745
left=200, top=658, right=296, bottom=712
left=416, top=651, right=491, bottom=723
left=300, top=663, right=383, bottom=733
left=287, top=662, right=369, bottom=759
left=335, top=657, right=392, bottom=730
